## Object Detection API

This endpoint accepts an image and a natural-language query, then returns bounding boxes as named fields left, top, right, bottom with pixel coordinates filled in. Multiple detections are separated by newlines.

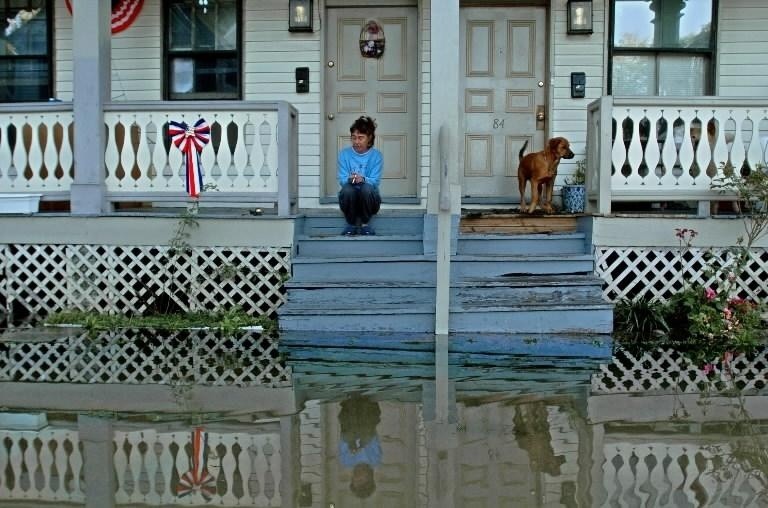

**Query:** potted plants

left=560, top=149, right=588, bottom=214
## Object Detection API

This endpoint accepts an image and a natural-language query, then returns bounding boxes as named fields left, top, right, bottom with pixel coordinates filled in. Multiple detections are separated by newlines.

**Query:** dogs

left=512, top=400, right=567, bottom=476
left=517, top=137, right=575, bottom=214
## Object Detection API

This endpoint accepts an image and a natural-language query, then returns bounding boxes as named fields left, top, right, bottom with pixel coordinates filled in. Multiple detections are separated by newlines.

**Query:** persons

left=336, top=399, right=383, bottom=500
left=335, top=115, right=385, bottom=235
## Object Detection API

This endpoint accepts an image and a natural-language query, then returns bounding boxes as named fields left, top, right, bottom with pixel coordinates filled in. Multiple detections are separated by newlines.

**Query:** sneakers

left=361, top=226, right=375, bottom=235
left=341, top=226, right=357, bottom=235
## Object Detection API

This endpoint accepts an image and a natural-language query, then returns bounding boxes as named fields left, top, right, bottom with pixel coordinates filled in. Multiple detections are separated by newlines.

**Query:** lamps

left=565, top=0, right=594, bottom=35
left=286, top=0, right=315, bottom=34
